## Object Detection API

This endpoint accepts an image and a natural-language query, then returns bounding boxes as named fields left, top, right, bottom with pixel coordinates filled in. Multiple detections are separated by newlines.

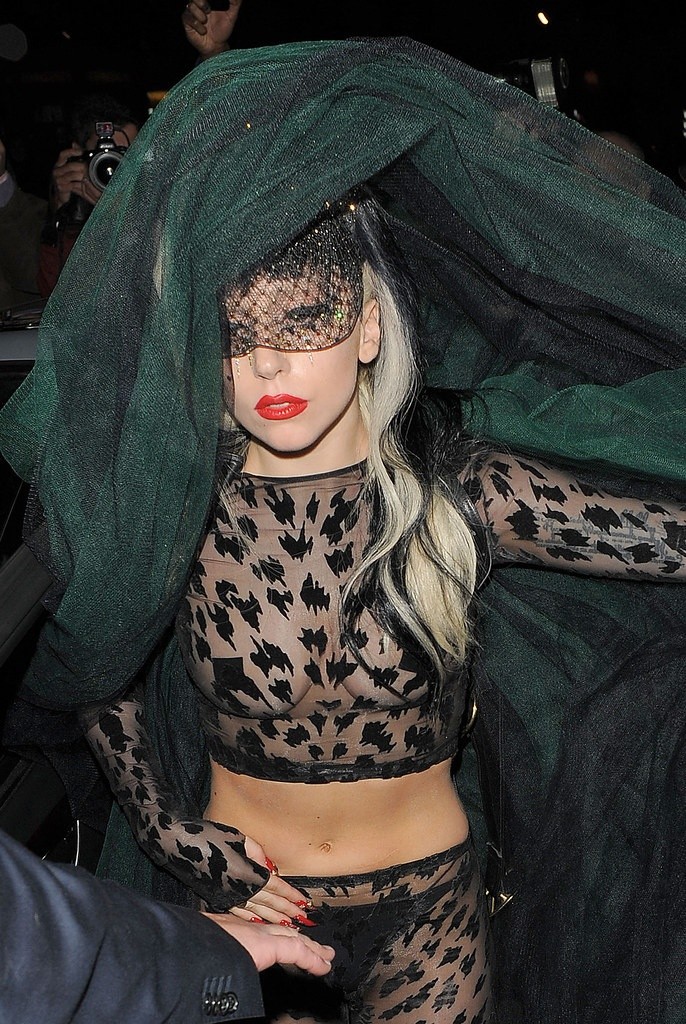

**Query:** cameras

left=67, top=121, right=129, bottom=194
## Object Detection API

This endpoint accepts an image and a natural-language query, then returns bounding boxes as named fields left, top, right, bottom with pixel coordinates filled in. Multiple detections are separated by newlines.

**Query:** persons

left=0, top=40, right=686, bottom=1024
left=0, top=831, right=334, bottom=1024
left=35, top=93, right=142, bottom=299
left=183, top=0, right=241, bottom=60
left=0, top=140, right=49, bottom=307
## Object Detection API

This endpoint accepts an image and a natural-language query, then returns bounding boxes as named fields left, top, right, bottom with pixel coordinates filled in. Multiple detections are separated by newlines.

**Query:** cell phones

left=207, top=0, right=230, bottom=11
